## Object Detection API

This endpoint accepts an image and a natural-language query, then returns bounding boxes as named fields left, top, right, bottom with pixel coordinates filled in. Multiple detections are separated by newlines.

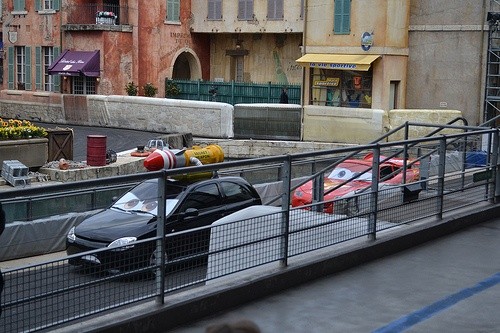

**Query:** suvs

left=64, top=171, right=263, bottom=283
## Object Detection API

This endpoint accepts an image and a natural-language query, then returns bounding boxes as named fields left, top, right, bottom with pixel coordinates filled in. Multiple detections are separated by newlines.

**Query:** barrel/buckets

left=86, top=135, right=108, bottom=167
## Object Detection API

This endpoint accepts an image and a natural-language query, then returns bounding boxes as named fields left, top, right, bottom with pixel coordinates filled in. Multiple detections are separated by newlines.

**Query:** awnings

left=295, top=53, right=382, bottom=72
left=47, top=49, right=100, bottom=77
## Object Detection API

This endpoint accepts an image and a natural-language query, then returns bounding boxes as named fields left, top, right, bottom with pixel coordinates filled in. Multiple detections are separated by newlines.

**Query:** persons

left=279, top=87, right=288, bottom=104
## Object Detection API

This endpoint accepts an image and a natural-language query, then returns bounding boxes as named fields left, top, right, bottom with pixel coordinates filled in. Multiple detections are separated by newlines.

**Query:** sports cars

left=290, top=157, right=425, bottom=214
